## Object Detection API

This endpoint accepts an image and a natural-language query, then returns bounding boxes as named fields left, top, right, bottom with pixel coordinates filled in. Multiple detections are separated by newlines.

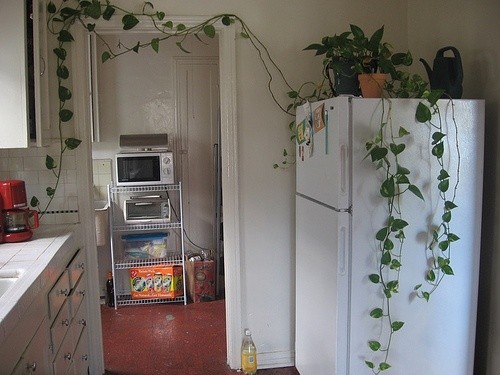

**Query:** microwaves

left=115, top=151, right=175, bottom=186
left=125, top=196, right=171, bottom=225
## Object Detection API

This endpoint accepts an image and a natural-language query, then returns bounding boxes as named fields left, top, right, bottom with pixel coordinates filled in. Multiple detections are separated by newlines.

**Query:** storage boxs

left=129, top=266, right=183, bottom=298
left=185, top=255, right=217, bottom=303
left=119, top=232, right=169, bottom=259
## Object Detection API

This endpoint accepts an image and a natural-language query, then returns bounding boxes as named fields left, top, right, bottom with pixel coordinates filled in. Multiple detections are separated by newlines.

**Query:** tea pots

left=419, top=45, right=463, bottom=99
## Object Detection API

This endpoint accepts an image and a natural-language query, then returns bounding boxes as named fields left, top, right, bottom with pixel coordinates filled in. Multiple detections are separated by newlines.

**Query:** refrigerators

left=294, top=98, right=485, bottom=375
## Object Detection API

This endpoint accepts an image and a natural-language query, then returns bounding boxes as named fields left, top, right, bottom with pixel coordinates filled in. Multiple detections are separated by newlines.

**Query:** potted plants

left=354, top=22, right=461, bottom=375
left=28, top=0, right=384, bottom=220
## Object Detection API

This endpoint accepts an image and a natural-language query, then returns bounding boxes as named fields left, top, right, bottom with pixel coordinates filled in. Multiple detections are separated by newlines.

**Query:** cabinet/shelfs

left=0, top=1, right=52, bottom=150
left=1, top=246, right=94, bottom=374
left=106, top=181, right=188, bottom=309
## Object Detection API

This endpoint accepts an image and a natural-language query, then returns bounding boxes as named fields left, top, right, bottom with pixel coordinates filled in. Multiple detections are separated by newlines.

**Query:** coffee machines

left=0, top=180, right=39, bottom=241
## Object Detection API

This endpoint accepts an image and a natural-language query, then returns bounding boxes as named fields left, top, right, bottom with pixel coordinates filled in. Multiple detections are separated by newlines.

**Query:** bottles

left=242, top=330, right=257, bottom=375
left=106, top=272, right=114, bottom=307
